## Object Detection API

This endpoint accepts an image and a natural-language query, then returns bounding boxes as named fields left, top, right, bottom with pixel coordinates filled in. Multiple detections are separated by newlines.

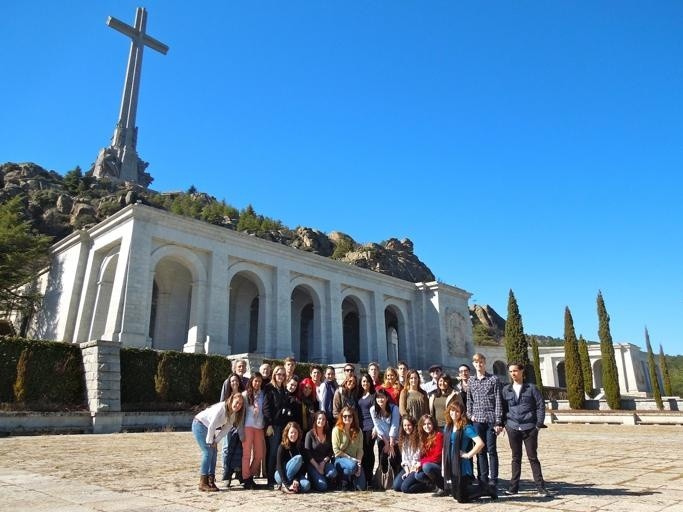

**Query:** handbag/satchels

left=373, top=448, right=395, bottom=491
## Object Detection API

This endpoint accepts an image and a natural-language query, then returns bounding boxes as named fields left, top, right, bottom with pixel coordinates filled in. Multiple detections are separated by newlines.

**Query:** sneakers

left=537, top=492, right=551, bottom=497
left=266, top=484, right=274, bottom=489
left=504, top=490, right=519, bottom=494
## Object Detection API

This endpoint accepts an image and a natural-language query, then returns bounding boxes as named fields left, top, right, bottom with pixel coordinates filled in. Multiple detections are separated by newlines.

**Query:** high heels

left=433, top=486, right=450, bottom=497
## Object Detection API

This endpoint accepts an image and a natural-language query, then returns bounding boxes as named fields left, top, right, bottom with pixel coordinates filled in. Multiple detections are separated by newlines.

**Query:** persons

left=191, top=352, right=553, bottom=504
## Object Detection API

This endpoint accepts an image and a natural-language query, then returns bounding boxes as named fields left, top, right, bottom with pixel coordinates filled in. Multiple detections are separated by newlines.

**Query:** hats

left=429, top=365, right=442, bottom=370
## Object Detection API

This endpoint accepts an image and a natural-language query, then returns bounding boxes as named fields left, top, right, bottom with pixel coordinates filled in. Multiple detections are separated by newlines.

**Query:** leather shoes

left=281, top=486, right=295, bottom=493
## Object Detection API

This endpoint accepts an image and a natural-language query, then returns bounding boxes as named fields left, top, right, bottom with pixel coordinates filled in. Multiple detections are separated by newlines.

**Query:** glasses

left=432, top=370, right=440, bottom=372
left=345, top=369, right=353, bottom=371
left=275, top=373, right=286, bottom=377
left=342, top=414, right=352, bottom=418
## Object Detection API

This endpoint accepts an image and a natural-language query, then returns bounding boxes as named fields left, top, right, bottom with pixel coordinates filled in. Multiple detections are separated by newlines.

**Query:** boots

left=207, top=475, right=219, bottom=491
left=199, top=475, right=216, bottom=491
left=481, top=484, right=498, bottom=500
left=225, top=475, right=257, bottom=489
